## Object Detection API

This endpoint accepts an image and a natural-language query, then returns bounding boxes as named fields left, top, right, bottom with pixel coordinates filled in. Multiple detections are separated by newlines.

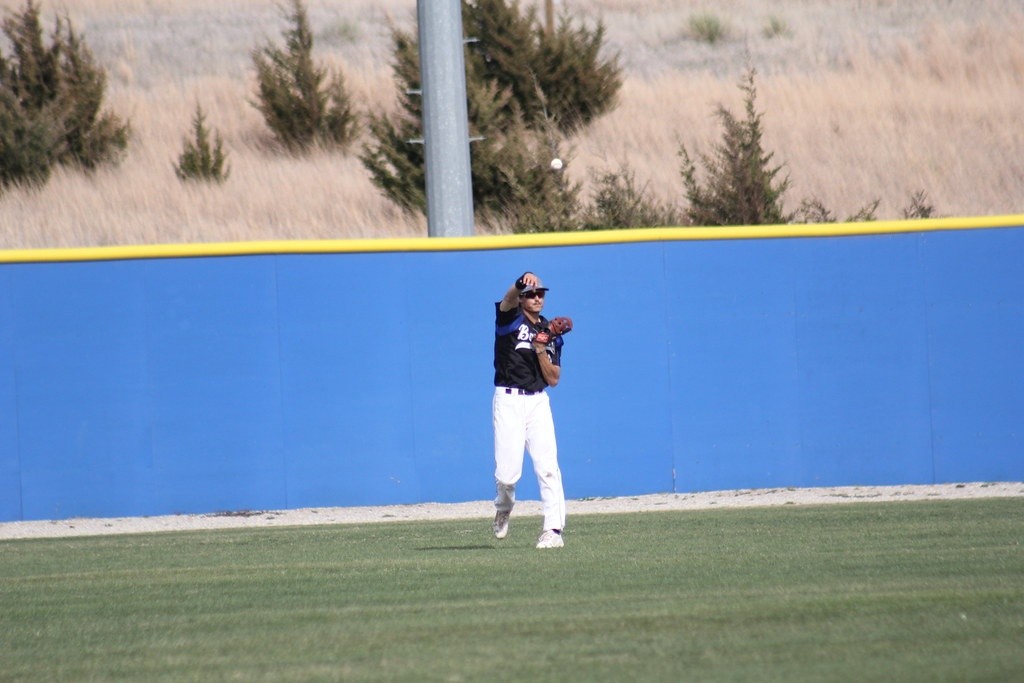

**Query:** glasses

left=521, top=290, right=545, bottom=299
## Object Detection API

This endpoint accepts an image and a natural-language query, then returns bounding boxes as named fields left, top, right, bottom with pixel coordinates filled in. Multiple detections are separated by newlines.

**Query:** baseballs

left=551, top=158, right=562, bottom=170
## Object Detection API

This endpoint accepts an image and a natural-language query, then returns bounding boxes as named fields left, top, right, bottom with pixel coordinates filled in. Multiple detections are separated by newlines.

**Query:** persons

left=491, top=272, right=565, bottom=548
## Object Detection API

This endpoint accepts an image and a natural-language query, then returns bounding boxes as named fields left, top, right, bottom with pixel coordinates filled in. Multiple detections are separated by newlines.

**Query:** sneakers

left=493, top=509, right=509, bottom=538
left=535, top=529, right=564, bottom=548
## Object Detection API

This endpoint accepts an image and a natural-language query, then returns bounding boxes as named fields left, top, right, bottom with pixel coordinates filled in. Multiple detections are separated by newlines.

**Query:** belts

left=506, top=388, right=543, bottom=395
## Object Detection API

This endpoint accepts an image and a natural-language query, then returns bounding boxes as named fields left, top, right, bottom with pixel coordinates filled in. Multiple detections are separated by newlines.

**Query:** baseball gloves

left=531, top=317, right=572, bottom=344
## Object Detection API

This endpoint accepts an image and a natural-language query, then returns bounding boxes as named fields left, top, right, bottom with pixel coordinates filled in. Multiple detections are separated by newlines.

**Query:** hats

left=521, top=281, right=549, bottom=294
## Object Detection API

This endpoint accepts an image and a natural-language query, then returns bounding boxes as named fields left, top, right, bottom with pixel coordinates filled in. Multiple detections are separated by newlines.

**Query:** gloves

left=532, top=339, right=546, bottom=353
